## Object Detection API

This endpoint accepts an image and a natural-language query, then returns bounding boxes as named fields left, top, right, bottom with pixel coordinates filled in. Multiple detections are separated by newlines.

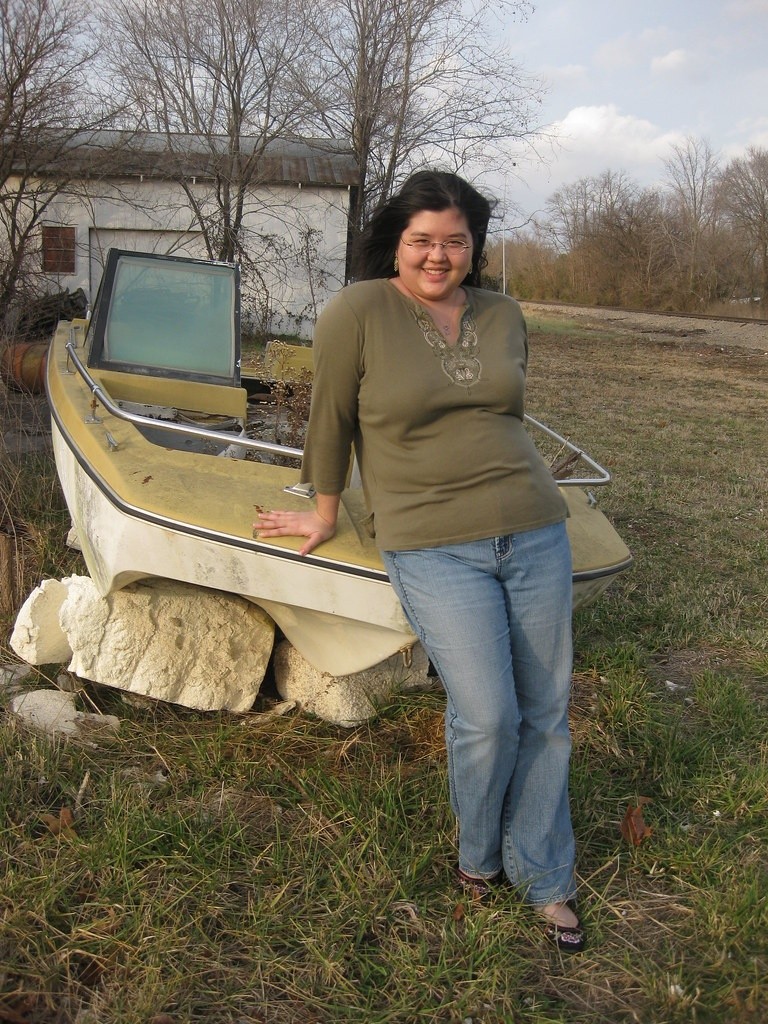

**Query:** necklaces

left=432, top=290, right=458, bottom=336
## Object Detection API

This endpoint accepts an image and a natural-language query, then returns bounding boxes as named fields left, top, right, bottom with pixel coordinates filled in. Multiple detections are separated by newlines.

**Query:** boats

left=44, top=248, right=636, bottom=680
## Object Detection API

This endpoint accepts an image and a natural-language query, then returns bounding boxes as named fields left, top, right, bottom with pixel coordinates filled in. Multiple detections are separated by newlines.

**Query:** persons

left=253, top=168, right=590, bottom=954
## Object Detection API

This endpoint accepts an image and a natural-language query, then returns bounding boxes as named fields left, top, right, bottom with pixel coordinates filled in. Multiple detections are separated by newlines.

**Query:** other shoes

left=459, top=871, right=503, bottom=904
left=526, top=901, right=587, bottom=953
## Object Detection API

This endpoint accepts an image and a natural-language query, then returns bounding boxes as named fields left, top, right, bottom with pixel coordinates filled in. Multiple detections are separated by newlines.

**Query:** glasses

left=400, top=236, right=473, bottom=255
